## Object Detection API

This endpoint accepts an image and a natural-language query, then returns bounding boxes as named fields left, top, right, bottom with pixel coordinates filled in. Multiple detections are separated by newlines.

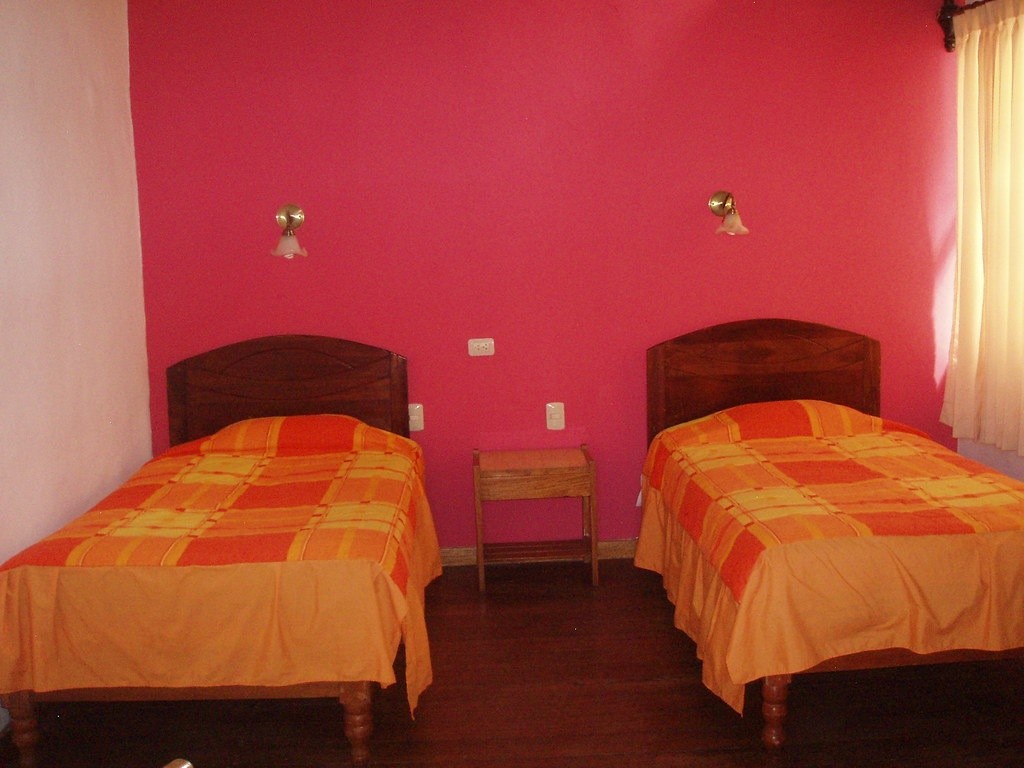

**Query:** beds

left=0, top=334, right=445, bottom=768
left=629, top=316, right=1024, bottom=752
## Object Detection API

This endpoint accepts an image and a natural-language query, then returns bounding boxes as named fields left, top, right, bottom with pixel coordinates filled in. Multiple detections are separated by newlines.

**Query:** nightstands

left=471, top=443, right=598, bottom=593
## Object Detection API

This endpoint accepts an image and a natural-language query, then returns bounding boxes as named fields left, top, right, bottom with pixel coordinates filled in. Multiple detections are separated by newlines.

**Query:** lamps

left=272, top=204, right=309, bottom=258
left=708, top=191, right=750, bottom=236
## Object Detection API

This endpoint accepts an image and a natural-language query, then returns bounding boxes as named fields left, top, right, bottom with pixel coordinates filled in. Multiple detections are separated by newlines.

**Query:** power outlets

left=467, top=338, right=495, bottom=356
